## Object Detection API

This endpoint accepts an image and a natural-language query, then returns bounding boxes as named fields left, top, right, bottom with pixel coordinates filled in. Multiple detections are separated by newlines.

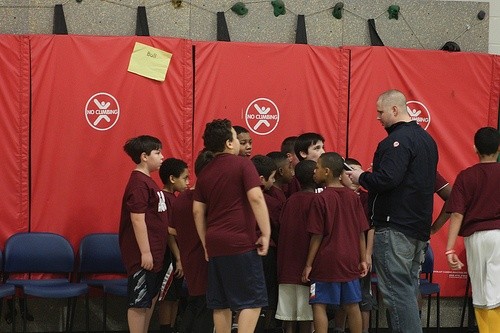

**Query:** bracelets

left=445, top=250, right=456, bottom=255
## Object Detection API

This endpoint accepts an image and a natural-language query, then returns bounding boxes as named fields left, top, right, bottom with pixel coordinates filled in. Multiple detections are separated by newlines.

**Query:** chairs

left=0, top=231, right=471, bottom=332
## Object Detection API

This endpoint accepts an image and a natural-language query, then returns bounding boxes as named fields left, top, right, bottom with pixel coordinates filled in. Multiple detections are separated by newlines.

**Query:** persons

left=301, top=152, right=371, bottom=333
left=344, top=89, right=438, bottom=333
left=192, top=119, right=272, bottom=333
left=446, top=127, right=500, bottom=333
left=118, top=135, right=168, bottom=333
left=155, top=126, right=451, bottom=333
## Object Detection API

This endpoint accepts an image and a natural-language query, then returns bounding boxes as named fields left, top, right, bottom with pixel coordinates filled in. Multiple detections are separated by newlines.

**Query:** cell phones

left=343, top=163, right=354, bottom=172
